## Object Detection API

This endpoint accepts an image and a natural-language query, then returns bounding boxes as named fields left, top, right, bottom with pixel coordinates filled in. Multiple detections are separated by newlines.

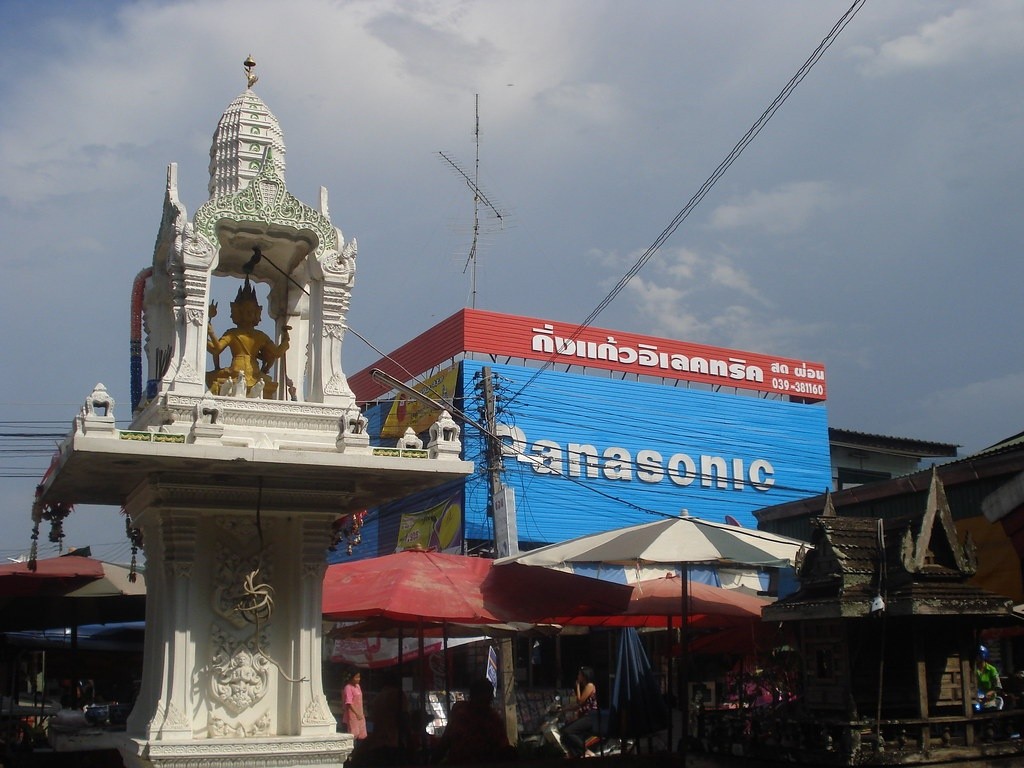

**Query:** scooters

left=973, top=689, right=1004, bottom=712
left=523, top=696, right=630, bottom=757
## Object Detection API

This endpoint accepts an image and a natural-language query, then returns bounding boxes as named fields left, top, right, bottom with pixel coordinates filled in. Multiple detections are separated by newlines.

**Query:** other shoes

left=581, top=753, right=596, bottom=759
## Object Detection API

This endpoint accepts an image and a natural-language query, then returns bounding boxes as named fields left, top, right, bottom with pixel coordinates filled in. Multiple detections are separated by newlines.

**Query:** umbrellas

left=493, top=510, right=814, bottom=768
left=0, top=550, right=147, bottom=711
left=321, top=553, right=561, bottom=768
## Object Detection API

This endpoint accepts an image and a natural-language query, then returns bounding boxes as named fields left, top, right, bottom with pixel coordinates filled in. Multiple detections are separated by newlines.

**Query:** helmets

left=979, top=645, right=988, bottom=660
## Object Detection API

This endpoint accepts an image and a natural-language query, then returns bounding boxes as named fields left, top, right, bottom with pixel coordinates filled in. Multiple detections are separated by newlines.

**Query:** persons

left=342, top=670, right=368, bottom=740
left=562, top=666, right=599, bottom=756
left=207, top=281, right=290, bottom=397
left=975, top=645, right=1004, bottom=711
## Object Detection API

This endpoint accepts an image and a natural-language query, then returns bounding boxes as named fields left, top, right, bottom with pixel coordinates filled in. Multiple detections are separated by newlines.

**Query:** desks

left=0, top=706, right=57, bottom=741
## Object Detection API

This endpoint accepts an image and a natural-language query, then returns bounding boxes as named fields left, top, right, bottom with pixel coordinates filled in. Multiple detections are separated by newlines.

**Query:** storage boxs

left=591, top=712, right=609, bottom=735
left=48, top=722, right=126, bottom=752
